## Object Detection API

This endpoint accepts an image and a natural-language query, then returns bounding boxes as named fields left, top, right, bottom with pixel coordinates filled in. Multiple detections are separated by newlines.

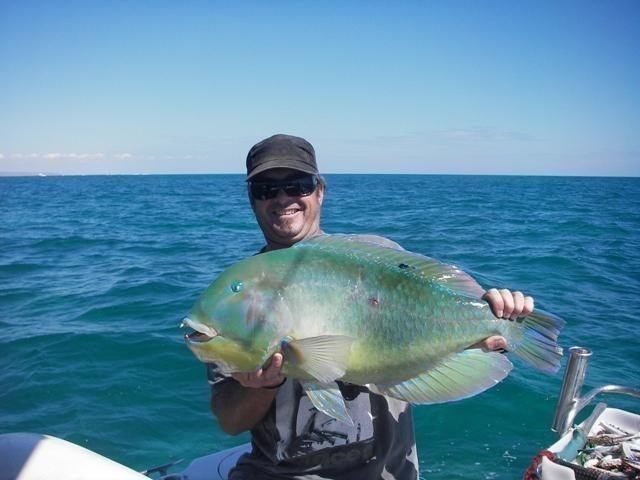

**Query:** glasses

left=247, top=177, right=316, bottom=201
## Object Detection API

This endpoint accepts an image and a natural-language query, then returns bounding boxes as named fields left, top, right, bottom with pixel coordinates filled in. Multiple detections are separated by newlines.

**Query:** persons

left=209, top=133, right=535, bottom=479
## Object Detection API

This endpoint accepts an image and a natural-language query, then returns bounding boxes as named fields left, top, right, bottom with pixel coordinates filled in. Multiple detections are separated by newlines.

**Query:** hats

left=246, top=134, right=320, bottom=178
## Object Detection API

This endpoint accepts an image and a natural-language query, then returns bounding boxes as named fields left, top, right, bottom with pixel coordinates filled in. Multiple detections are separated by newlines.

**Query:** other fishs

left=183, top=234, right=568, bottom=429
